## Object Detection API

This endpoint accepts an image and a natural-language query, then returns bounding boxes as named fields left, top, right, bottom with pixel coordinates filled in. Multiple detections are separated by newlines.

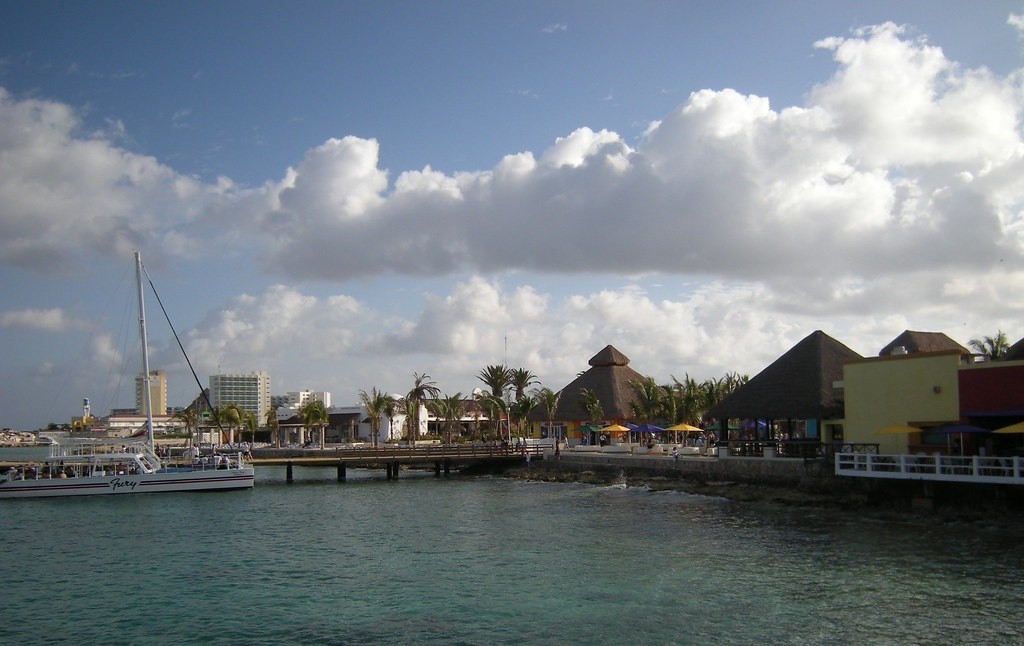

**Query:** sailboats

left=0, top=252, right=254, bottom=498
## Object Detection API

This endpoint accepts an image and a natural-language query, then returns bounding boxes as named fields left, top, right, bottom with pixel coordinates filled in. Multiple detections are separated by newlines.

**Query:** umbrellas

left=923, top=423, right=988, bottom=474
left=623, top=422, right=666, bottom=432
left=570, top=424, right=600, bottom=445
left=871, top=422, right=923, bottom=455
left=598, top=424, right=630, bottom=442
left=701, top=423, right=742, bottom=430
left=664, top=423, right=704, bottom=444
left=989, top=421, right=1024, bottom=433
left=738, top=420, right=770, bottom=428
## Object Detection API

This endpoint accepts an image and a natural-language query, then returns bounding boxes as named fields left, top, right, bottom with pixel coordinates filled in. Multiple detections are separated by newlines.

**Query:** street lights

left=506, top=407, right=511, bottom=442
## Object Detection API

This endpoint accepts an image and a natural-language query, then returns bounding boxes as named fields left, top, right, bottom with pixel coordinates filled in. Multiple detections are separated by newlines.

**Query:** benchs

left=574, top=444, right=705, bottom=454
left=305, top=443, right=316, bottom=449
left=192, top=463, right=227, bottom=469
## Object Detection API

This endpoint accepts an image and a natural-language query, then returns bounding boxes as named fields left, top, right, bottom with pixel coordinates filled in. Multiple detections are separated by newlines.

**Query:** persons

left=709, top=431, right=715, bottom=447
left=777, top=430, right=800, bottom=439
left=672, top=447, right=679, bottom=462
left=599, top=433, right=606, bottom=447
left=564, top=435, right=569, bottom=448
left=695, top=437, right=703, bottom=447
left=516, top=437, right=528, bottom=455
